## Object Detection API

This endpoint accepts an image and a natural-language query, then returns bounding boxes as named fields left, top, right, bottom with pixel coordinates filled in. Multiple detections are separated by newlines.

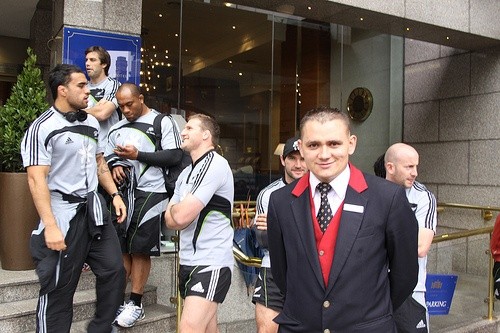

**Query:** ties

left=315, top=182, right=332, bottom=232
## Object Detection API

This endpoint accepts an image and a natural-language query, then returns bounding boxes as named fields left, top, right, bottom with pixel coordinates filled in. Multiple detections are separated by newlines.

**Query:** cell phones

left=117, top=146, right=124, bottom=152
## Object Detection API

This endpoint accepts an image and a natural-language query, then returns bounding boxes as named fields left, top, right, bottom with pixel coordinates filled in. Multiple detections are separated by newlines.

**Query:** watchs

left=111, top=192, right=123, bottom=199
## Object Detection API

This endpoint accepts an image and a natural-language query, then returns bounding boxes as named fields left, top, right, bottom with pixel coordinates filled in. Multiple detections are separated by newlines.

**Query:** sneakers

left=112, top=300, right=146, bottom=328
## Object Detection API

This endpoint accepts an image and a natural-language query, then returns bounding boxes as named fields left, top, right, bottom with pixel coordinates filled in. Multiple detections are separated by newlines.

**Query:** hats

left=283, top=138, right=302, bottom=159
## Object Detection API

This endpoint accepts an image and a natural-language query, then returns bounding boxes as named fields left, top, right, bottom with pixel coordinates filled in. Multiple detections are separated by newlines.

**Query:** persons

left=373, top=155, right=387, bottom=180
left=384, top=143, right=438, bottom=333
left=252, top=137, right=309, bottom=333
left=266, top=107, right=419, bottom=333
left=231, top=152, right=270, bottom=201
left=156, top=97, right=193, bottom=200
left=79, top=46, right=125, bottom=157
left=490, top=214, right=500, bottom=301
left=103, top=82, right=187, bottom=329
left=21, top=63, right=127, bottom=333
left=165, top=112, right=235, bottom=333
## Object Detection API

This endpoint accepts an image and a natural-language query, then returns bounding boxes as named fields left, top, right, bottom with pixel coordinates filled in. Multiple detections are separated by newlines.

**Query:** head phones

left=53, top=104, right=87, bottom=122
